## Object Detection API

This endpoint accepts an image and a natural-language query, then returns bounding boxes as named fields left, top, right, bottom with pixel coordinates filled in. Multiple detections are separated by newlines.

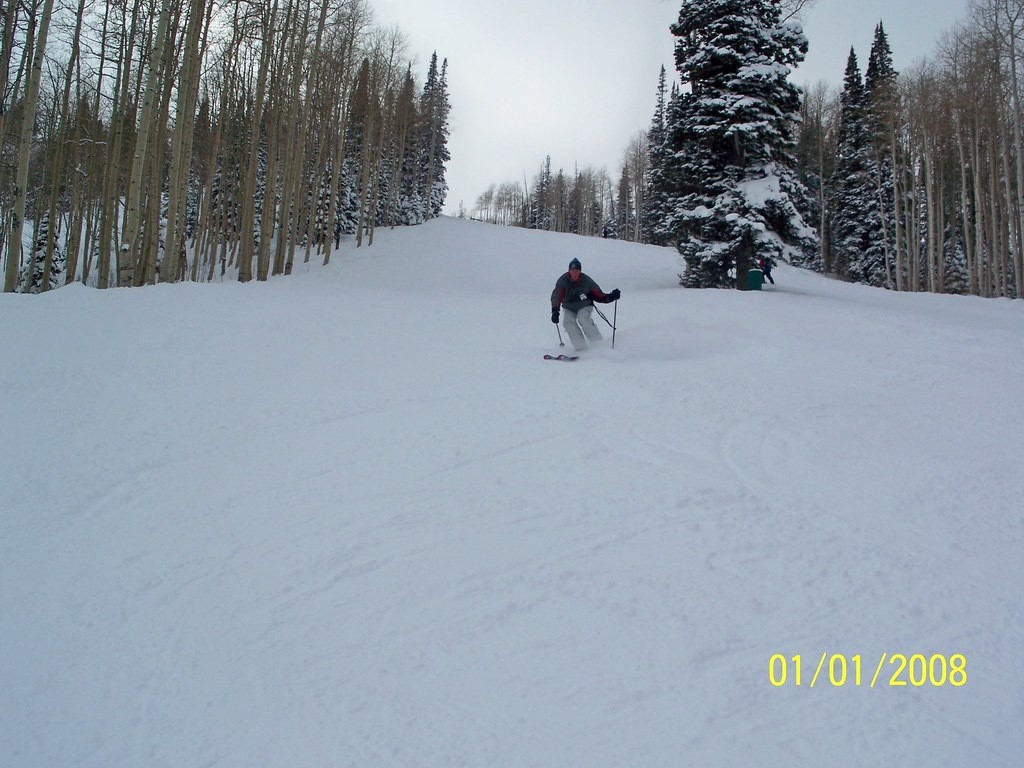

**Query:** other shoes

left=762, top=281, right=765, bottom=282
left=772, top=281, right=774, bottom=284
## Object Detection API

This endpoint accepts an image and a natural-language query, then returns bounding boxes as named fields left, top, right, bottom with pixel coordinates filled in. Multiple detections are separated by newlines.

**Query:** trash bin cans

left=746, top=269, right=763, bottom=292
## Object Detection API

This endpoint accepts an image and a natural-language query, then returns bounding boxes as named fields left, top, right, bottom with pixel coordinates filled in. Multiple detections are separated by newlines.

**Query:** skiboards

left=544, top=354, right=582, bottom=361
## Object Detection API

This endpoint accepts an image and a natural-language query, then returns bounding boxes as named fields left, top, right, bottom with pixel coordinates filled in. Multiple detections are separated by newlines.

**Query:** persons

left=751, top=258, right=775, bottom=284
left=551, top=258, right=621, bottom=355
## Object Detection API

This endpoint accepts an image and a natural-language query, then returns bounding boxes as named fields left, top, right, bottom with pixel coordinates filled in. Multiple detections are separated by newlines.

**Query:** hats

left=569, top=258, right=581, bottom=270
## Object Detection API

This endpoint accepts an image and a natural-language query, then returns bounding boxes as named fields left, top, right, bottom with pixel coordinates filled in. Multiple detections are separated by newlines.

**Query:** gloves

left=608, top=289, right=621, bottom=302
left=551, top=307, right=560, bottom=323
